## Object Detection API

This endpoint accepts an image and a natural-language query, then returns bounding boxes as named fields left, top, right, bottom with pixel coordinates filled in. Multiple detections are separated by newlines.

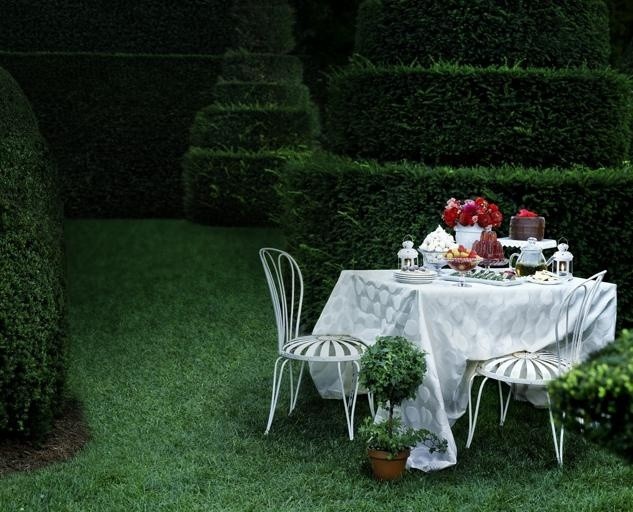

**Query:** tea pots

left=508, top=236, right=558, bottom=277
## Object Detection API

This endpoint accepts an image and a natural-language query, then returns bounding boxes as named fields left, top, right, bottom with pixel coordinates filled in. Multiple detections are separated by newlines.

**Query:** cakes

left=508, top=209, right=545, bottom=240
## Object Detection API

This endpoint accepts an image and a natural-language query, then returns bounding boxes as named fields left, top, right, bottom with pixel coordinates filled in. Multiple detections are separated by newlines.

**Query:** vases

left=454, top=224, right=494, bottom=246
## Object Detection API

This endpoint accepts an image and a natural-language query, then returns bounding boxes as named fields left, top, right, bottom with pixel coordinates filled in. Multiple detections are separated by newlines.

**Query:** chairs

left=258, top=248, right=375, bottom=441
left=465, top=269, right=610, bottom=467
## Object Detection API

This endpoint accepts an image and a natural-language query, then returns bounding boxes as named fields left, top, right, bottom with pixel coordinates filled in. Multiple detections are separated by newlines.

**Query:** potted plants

left=347, top=338, right=446, bottom=480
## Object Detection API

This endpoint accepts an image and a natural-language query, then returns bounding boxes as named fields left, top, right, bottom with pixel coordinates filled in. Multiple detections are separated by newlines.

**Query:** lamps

left=396, top=232, right=418, bottom=269
left=550, top=236, right=573, bottom=280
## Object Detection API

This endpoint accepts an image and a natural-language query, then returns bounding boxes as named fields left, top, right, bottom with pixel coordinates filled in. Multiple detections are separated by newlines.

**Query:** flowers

left=444, top=197, right=500, bottom=228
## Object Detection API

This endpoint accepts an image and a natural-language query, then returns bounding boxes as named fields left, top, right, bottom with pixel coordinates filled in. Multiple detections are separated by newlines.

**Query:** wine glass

left=444, top=257, right=483, bottom=287
left=418, top=249, right=455, bottom=278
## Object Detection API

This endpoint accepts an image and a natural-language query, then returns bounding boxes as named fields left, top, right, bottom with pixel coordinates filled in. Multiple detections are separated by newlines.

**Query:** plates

left=394, top=269, right=437, bottom=284
left=523, top=272, right=569, bottom=285
left=443, top=267, right=522, bottom=286
